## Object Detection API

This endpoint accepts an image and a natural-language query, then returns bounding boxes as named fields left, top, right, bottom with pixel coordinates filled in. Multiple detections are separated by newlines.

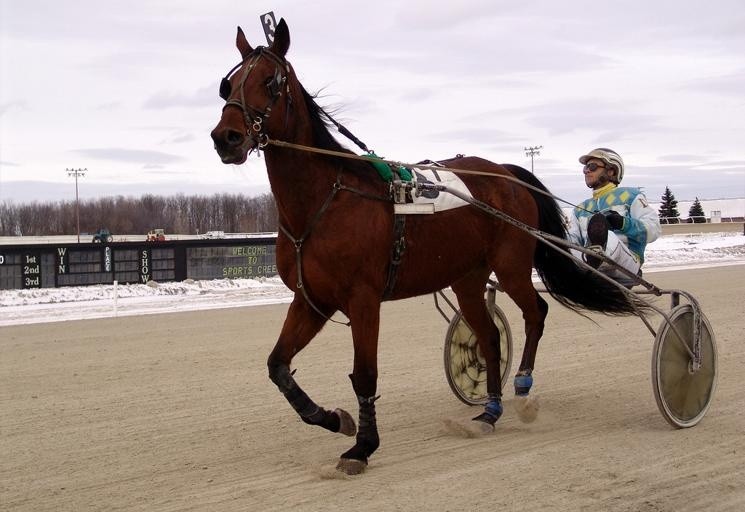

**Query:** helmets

left=579, top=148, right=624, bottom=184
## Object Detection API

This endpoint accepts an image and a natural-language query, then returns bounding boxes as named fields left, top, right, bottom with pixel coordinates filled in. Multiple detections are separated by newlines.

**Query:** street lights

left=66, top=169, right=87, bottom=242
left=524, top=145, right=541, bottom=175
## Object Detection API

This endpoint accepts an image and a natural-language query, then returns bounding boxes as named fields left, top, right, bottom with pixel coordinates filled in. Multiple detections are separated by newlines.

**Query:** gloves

left=606, top=210, right=623, bottom=230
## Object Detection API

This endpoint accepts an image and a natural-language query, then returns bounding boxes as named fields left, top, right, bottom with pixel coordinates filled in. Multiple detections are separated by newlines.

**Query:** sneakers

left=587, top=214, right=607, bottom=269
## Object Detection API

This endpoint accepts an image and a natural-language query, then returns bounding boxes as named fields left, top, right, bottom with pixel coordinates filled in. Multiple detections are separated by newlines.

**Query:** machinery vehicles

left=92, top=229, right=113, bottom=243
left=146, top=229, right=165, bottom=241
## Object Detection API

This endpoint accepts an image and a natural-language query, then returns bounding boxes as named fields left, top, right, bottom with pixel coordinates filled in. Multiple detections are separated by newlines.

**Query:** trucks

left=203, top=231, right=224, bottom=239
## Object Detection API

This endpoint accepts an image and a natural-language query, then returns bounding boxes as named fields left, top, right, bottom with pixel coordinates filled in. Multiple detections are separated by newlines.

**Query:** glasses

left=583, top=163, right=607, bottom=174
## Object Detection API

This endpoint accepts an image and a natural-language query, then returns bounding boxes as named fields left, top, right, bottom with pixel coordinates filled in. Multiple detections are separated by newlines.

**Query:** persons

left=563, top=148, right=662, bottom=291
